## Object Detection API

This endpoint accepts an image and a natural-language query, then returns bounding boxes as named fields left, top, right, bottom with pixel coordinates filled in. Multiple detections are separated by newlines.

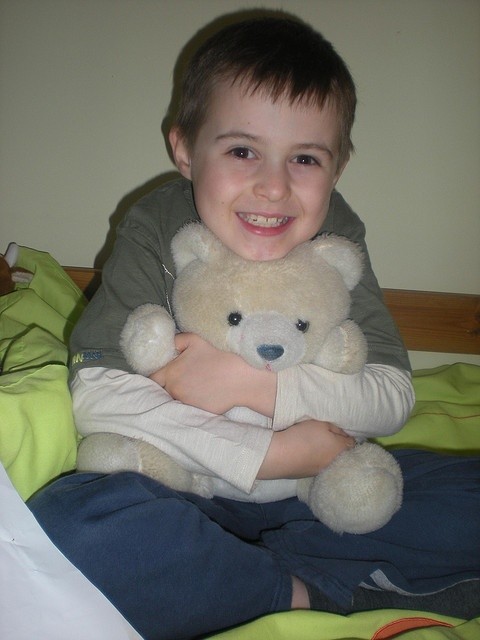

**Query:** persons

left=24, top=6, right=480, bottom=640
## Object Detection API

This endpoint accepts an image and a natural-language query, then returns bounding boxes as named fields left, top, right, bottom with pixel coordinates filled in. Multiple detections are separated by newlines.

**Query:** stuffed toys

left=76, top=219, right=405, bottom=534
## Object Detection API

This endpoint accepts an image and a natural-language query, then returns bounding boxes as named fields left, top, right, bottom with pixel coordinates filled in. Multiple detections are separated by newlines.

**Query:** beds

left=1, top=266, right=479, bottom=640
